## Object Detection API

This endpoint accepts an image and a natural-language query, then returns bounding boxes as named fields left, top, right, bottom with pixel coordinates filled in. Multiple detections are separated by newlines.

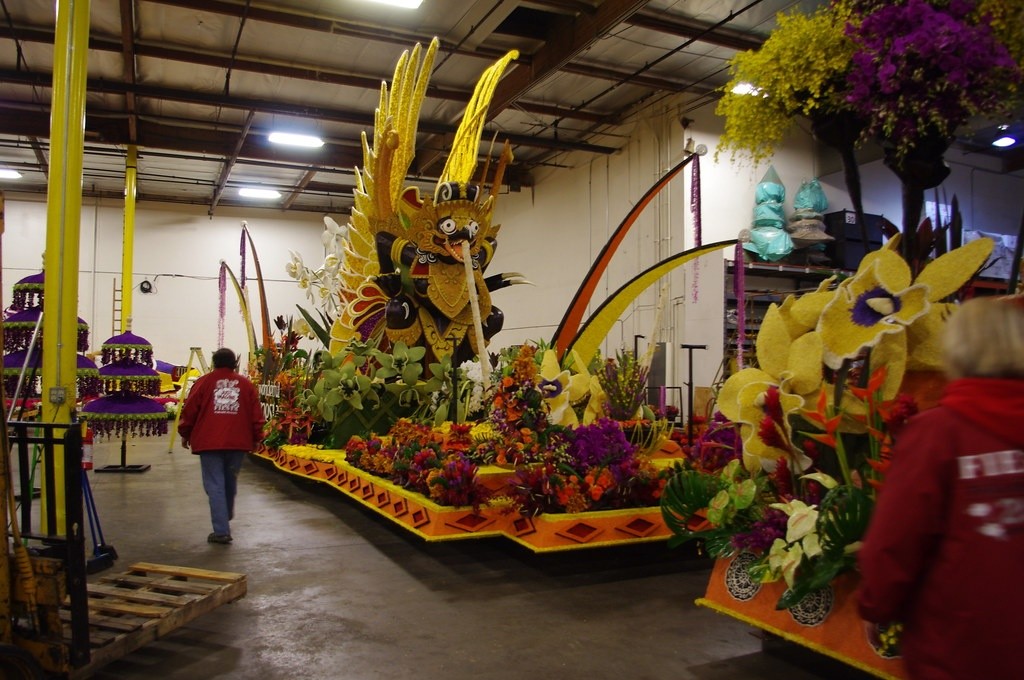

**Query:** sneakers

left=208, top=533, right=231, bottom=542
left=227, top=504, right=233, bottom=520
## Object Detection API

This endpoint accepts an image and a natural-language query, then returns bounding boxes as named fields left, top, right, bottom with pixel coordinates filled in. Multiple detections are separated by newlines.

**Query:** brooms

left=82, top=468, right=118, bottom=574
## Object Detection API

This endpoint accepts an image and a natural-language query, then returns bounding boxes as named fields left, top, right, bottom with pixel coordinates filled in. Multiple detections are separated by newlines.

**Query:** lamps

left=239, top=189, right=281, bottom=199
left=0, top=169, right=23, bottom=179
left=368, top=0, right=424, bottom=10
left=267, top=132, right=325, bottom=148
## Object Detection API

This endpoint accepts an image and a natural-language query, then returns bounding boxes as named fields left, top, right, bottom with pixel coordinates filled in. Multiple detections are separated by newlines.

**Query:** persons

left=862, top=292, right=1024, bottom=680
left=178, top=348, right=264, bottom=543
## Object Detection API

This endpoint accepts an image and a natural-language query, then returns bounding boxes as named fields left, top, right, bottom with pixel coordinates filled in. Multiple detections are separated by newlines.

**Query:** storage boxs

left=823, top=208, right=885, bottom=271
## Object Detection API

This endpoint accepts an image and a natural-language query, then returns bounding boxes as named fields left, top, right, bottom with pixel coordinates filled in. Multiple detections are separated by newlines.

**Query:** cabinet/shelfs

left=721, top=252, right=856, bottom=381
